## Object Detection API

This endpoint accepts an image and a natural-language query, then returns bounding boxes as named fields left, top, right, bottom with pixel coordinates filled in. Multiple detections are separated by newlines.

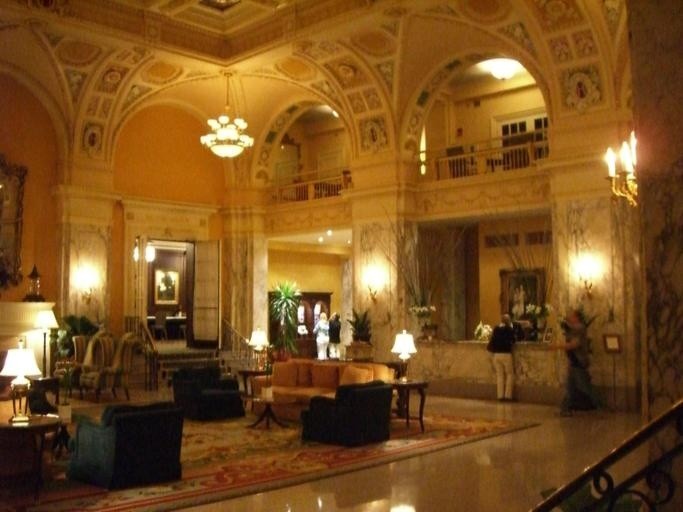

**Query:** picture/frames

left=0, top=152, right=29, bottom=287
left=154, top=267, right=180, bottom=306
left=500, top=267, right=546, bottom=323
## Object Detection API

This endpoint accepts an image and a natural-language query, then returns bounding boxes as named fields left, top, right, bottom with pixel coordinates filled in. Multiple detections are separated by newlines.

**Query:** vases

left=536, top=316, right=546, bottom=340
left=410, top=306, right=437, bottom=315
left=422, top=315, right=434, bottom=341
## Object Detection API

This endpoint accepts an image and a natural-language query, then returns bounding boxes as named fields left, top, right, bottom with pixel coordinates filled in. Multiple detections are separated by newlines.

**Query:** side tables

left=237, top=367, right=265, bottom=400
left=393, top=381, right=430, bottom=435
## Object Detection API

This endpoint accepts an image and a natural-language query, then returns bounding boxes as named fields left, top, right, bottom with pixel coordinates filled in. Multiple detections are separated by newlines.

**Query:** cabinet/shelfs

left=0, top=299, right=58, bottom=382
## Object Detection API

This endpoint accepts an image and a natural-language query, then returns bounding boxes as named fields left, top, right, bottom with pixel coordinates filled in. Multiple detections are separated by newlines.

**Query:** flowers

left=526, top=303, right=554, bottom=318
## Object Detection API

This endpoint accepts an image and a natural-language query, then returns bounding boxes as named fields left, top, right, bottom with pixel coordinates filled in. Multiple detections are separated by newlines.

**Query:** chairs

left=53, top=329, right=138, bottom=406
left=341, top=167, right=352, bottom=189
left=293, top=170, right=311, bottom=202
left=66, top=400, right=185, bottom=491
left=446, top=131, right=549, bottom=178
left=301, top=380, right=394, bottom=448
left=171, top=366, right=244, bottom=419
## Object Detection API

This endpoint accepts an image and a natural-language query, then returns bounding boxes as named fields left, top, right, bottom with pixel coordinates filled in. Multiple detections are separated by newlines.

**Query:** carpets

left=0, top=379, right=543, bottom=512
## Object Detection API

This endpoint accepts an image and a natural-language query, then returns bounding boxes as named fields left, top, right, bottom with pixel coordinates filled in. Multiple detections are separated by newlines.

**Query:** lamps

left=33, top=308, right=60, bottom=380
left=200, top=71, right=256, bottom=159
left=389, top=329, right=418, bottom=383
left=248, top=326, right=268, bottom=370
left=0, top=346, right=43, bottom=422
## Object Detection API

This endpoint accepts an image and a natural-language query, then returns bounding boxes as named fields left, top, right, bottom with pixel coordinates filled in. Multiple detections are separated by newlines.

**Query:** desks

left=315, top=181, right=340, bottom=197
left=147, top=315, right=187, bottom=320
left=150, top=311, right=169, bottom=340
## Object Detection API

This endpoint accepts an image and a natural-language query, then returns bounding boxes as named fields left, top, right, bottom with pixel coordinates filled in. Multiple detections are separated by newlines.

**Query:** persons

left=326, top=312, right=341, bottom=360
left=312, top=312, right=329, bottom=360
left=549, top=309, right=604, bottom=417
left=517, top=282, right=527, bottom=319
left=485, top=313, right=516, bottom=401
left=512, top=285, right=519, bottom=316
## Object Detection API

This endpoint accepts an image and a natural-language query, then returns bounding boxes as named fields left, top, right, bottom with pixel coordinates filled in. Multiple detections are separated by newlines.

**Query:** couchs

left=251, top=356, right=395, bottom=421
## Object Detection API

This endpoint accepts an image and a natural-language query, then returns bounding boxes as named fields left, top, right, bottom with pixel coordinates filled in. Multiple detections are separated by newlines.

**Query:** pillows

left=272, top=358, right=375, bottom=387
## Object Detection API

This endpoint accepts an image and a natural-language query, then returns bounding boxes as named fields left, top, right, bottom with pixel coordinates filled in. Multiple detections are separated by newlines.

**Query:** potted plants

left=346, top=306, right=374, bottom=362
left=269, top=280, right=302, bottom=361
